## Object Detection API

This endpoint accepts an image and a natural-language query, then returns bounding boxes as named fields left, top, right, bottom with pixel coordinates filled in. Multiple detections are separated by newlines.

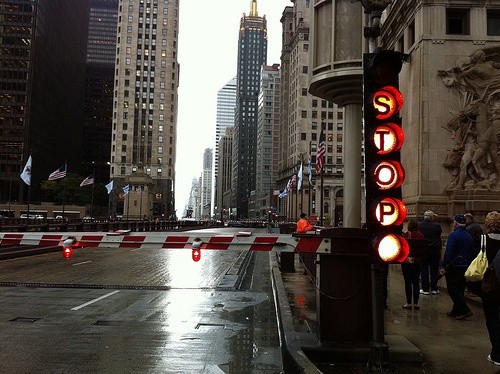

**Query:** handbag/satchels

left=402, top=231, right=415, bottom=264
left=464, top=234, right=489, bottom=282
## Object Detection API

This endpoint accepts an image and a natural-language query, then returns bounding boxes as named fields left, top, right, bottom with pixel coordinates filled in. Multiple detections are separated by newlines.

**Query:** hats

left=301, top=213, right=307, bottom=218
left=449, top=214, right=465, bottom=224
left=424, top=210, right=438, bottom=216
left=464, top=213, right=472, bottom=217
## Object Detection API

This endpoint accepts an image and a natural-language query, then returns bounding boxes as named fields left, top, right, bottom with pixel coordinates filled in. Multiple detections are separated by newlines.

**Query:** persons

left=453, top=50, right=500, bottom=82
left=474, top=211, right=500, bottom=366
left=401, top=219, right=425, bottom=310
left=418, top=210, right=442, bottom=295
left=440, top=214, right=473, bottom=320
left=297, top=212, right=314, bottom=233
left=465, top=214, right=483, bottom=298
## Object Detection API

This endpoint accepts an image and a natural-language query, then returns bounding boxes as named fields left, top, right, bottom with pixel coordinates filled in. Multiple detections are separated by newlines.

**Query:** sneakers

left=431, top=290, right=439, bottom=294
left=420, top=289, right=429, bottom=295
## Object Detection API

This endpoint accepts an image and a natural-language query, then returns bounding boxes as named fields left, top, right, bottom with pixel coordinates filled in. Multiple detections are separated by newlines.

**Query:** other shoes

left=402, top=304, right=412, bottom=309
left=487, top=354, right=500, bottom=366
left=414, top=305, right=420, bottom=310
left=447, top=312, right=460, bottom=316
left=456, top=311, right=473, bottom=319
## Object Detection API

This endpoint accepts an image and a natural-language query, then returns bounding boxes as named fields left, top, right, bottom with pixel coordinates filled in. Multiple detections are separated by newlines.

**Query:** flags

left=105, top=179, right=114, bottom=194
left=137, top=186, right=144, bottom=196
left=279, top=175, right=296, bottom=199
left=48, top=164, right=67, bottom=181
left=307, top=153, right=315, bottom=186
left=20, top=154, right=32, bottom=186
left=123, top=183, right=130, bottom=197
left=316, top=134, right=326, bottom=177
left=297, top=161, right=304, bottom=193
left=80, top=174, right=95, bottom=187
